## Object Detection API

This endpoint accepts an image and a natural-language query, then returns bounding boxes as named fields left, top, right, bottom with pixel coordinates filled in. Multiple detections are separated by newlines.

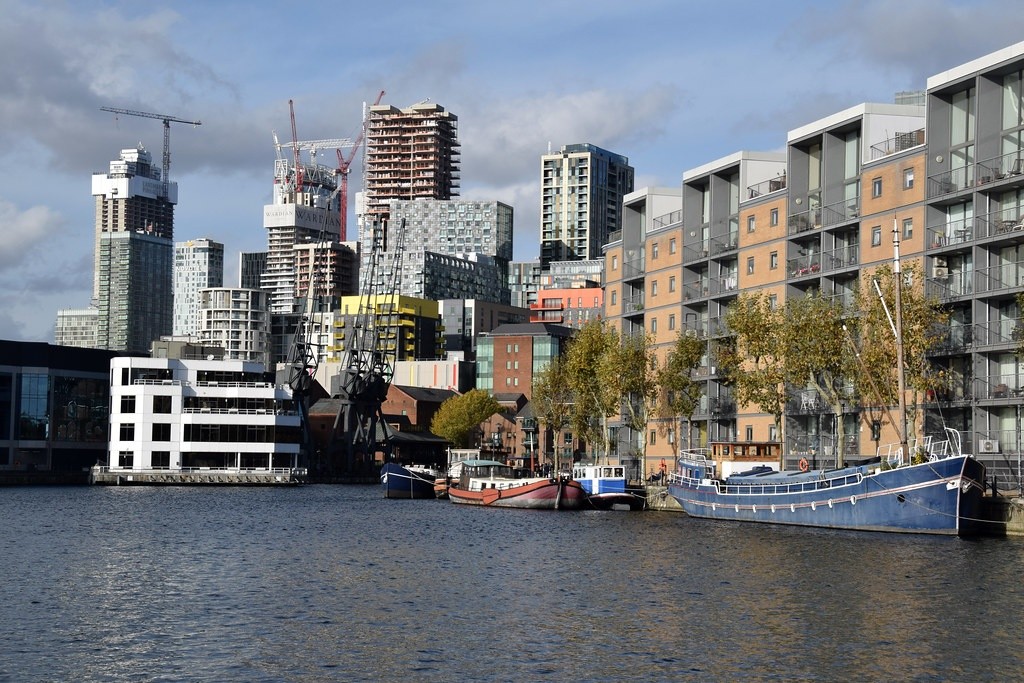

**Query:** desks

left=1002, top=220, right=1019, bottom=233
left=954, top=230, right=965, bottom=242
left=1011, top=389, right=1023, bottom=397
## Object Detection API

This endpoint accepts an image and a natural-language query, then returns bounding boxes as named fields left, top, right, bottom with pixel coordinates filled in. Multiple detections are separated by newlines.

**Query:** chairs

left=992, top=384, right=1007, bottom=399
left=963, top=226, right=973, bottom=242
left=1007, top=159, right=1024, bottom=177
left=994, top=214, right=1024, bottom=235
left=990, top=168, right=1006, bottom=181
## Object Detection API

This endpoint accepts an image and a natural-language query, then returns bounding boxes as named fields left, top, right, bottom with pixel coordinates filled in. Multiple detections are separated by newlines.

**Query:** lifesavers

left=800, top=460, right=809, bottom=471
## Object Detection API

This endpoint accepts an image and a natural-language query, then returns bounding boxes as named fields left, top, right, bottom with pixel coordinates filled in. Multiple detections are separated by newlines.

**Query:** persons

left=751, top=450, right=756, bottom=456
left=535, top=460, right=555, bottom=478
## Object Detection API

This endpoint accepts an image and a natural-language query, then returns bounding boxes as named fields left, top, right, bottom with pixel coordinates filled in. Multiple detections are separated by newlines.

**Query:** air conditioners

left=932, top=268, right=948, bottom=279
left=933, top=256, right=947, bottom=267
left=979, top=439, right=999, bottom=453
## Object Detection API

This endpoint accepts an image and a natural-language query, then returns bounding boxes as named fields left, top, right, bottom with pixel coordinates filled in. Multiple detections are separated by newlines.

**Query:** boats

left=569, top=461, right=648, bottom=512
left=446, top=459, right=585, bottom=512
left=379, top=458, right=438, bottom=500
left=432, top=475, right=460, bottom=500
left=663, top=209, right=988, bottom=536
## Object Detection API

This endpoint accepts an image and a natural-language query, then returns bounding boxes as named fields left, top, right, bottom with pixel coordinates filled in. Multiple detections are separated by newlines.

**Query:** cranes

left=99, top=104, right=202, bottom=182
left=268, top=87, right=387, bottom=242
